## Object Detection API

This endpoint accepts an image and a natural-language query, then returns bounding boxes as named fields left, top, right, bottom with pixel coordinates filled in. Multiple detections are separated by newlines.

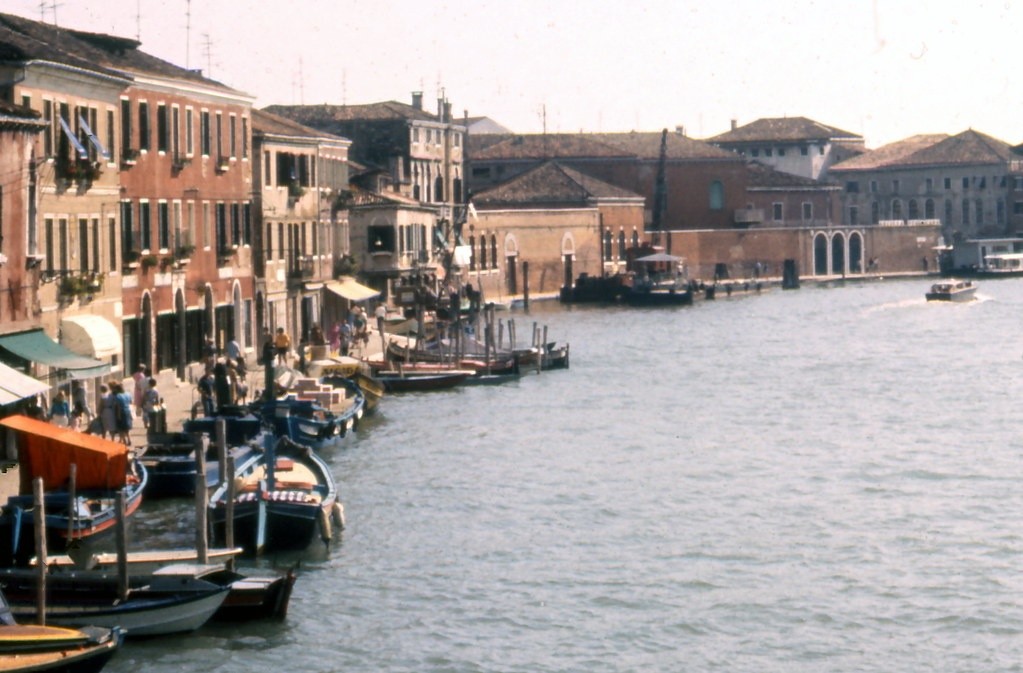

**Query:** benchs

left=264, top=460, right=293, bottom=471
left=233, top=481, right=326, bottom=493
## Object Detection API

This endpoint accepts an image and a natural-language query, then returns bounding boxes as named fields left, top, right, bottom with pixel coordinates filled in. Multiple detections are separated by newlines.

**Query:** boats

left=1, top=451, right=148, bottom=549
left=138, top=405, right=283, bottom=499
left=0, top=569, right=233, bottom=640
left=364, top=334, right=569, bottom=394
left=208, top=435, right=345, bottom=555
left=0, top=623, right=129, bottom=673
left=925, top=279, right=978, bottom=302
left=249, top=374, right=367, bottom=449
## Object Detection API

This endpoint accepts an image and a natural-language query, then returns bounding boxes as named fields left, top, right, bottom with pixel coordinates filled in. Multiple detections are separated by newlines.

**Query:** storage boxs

left=288, top=379, right=346, bottom=408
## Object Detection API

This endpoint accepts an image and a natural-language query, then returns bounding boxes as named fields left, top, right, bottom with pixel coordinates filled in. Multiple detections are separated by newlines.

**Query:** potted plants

left=58, top=273, right=106, bottom=303
left=119, top=149, right=140, bottom=165
left=121, top=251, right=142, bottom=268
left=175, top=244, right=197, bottom=265
left=288, top=185, right=305, bottom=203
left=217, top=247, right=238, bottom=263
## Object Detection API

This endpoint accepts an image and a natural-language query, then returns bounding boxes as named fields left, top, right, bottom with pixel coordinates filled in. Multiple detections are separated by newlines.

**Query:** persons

left=261, top=325, right=291, bottom=365
left=28, top=363, right=160, bottom=446
left=677, top=261, right=683, bottom=275
left=924, top=256, right=927, bottom=269
left=375, top=302, right=387, bottom=329
left=312, top=303, right=368, bottom=357
left=196, top=333, right=247, bottom=418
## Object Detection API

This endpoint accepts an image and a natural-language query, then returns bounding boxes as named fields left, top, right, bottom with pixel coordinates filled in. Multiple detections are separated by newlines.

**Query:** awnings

left=61, top=314, right=122, bottom=358
left=0, top=361, right=52, bottom=405
left=326, top=279, right=381, bottom=302
left=0, top=330, right=112, bottom=379
